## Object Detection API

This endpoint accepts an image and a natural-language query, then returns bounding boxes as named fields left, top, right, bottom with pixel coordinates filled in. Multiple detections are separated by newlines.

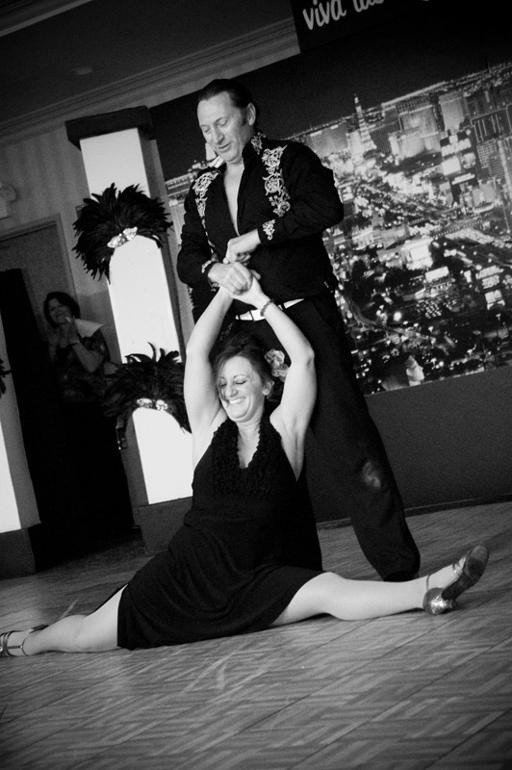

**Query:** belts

left=233, top=298, right=306, bottom=323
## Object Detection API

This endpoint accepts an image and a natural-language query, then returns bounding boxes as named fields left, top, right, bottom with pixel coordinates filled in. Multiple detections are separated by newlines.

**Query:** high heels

left=424, top=546, right=489, bottom=617
left=1, top=624, right=49, bottom=656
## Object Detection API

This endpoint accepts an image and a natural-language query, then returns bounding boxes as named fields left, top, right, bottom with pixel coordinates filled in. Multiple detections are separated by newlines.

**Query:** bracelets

left=260, top=298, right=275, bottom=316
left=70, top=338, right=80, bottom=348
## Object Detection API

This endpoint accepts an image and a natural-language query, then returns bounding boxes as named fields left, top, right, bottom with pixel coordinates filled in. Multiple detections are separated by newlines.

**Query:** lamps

left=0, top=181, right=17, bottom=219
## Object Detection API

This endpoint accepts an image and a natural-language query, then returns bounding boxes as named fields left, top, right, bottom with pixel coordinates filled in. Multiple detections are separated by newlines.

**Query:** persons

left=0, top=282, right=488, bottom=658
left=176, top=76, right=421, bottom=582
left=42, top=291, right=123, bottom=490
